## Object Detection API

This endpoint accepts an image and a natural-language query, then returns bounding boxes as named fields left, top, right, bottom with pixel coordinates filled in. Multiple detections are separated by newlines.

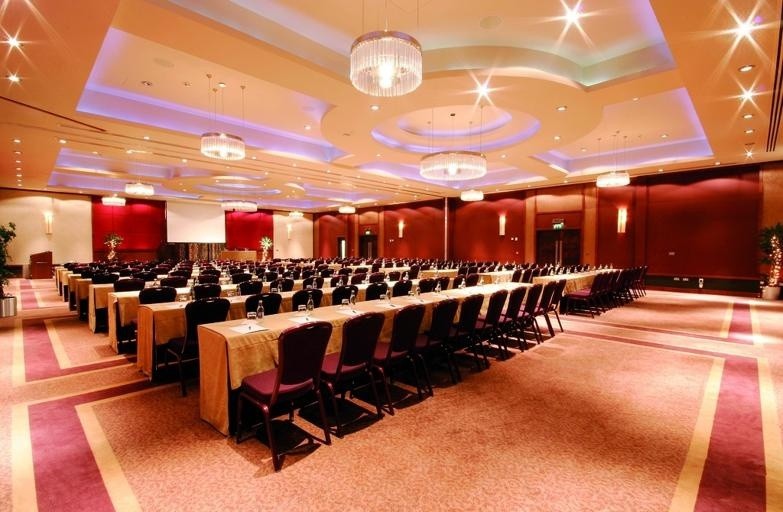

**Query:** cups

left=408, top=291, right=415, bottom=300
left=247, top=312, right=256, bottom=323
left=342, top=299, right=349, bottom=310
left=380, top=294, right=386, bottom=303
left=297, top=304, right=306, bottom=316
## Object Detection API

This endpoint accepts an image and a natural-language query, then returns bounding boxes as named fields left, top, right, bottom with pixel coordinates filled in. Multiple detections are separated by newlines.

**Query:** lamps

left=201, top=73, right=246, bottom=161
left=124, top=182, right=155, bottom=197
left=419, top=104, right=487, bottom=181
left=350, top=0, right=421, bottom=98
left=220, top=200, right=258, bottom=214
left=596, top=131, right=629, bottom=188
left=44, top=209, right=53, bottom=234
left=617, top=207, right=626, bottom=233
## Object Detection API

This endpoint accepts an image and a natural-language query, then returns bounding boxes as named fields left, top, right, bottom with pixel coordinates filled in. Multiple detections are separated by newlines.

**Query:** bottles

left=306, top=294, right=314, bottom=315
left=85, top=257, right=501, bottom=324
left=386, top=288, right=392, bottom=303
left=257, top=299, right=264, bottom=322
left=501, top=260, right=614, bottom=278
left=350, top=290, right=356, bottom=306
left=415, top=285, right=421, bottom=299
left=437, top=281, right=441, bottom=294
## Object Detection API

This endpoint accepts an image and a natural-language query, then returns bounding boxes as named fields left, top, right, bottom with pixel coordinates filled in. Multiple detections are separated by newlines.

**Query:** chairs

left=56, top=258, right=648, bottom=471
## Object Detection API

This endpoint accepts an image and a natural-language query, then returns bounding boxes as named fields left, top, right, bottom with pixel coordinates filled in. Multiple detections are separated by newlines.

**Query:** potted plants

left=757, top=222, right=783, bottom=300
left=0, top=222, right=17, bottom=317
